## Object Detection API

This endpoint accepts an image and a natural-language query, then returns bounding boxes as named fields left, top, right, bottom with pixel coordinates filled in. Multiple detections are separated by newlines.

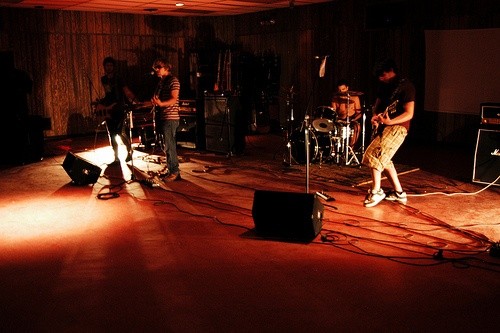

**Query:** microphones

left=145, top=71, right=154, bottom=77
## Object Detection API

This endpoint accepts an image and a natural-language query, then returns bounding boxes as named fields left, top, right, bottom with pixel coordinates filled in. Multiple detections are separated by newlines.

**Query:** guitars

left=370, top=99, right=398, bottom=140
left=95, top=103, right=117, bottom=122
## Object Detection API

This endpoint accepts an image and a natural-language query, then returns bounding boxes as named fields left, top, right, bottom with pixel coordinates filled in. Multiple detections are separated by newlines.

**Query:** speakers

left=205, top=95, right=237, bottom=155
left=251, top=190, right=324, bottom=239
left=62, top=151, right=102, bottom=185
left=174, top=114, right=204, bottom=150
left=472, top=129, right=500, bottom=187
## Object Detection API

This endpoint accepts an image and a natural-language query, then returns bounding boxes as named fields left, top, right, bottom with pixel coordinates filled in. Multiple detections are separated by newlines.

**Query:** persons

left=329, top=81, right=362, bottom=158
left=361, top=59, right=415, bottom=208
left=102, top=57, right=139, bottom=168
left=151, top=58, right=180, bottom=182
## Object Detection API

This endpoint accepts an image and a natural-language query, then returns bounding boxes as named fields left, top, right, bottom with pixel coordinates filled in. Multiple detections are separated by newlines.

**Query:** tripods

left=109, top=77, right=152, bottom=190
left=325, top=95, right=368, bottom=169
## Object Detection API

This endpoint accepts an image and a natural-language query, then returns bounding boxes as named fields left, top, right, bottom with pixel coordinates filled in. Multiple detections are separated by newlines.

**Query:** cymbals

left=334, top=90, right=365, bottom=96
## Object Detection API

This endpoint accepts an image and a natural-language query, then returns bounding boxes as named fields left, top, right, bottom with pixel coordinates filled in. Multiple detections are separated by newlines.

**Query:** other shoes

left=386, top=190, right=407, bottom=200
left=363, top=187, right=386, bottom=207
left=163, top=171, right=180, bottom=182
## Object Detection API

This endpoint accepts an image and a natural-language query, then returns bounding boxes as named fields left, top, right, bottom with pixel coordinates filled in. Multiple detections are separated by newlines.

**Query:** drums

left=311, top=105, right=337, bottom=132
left=290, top=126, right=333, bottom=166
